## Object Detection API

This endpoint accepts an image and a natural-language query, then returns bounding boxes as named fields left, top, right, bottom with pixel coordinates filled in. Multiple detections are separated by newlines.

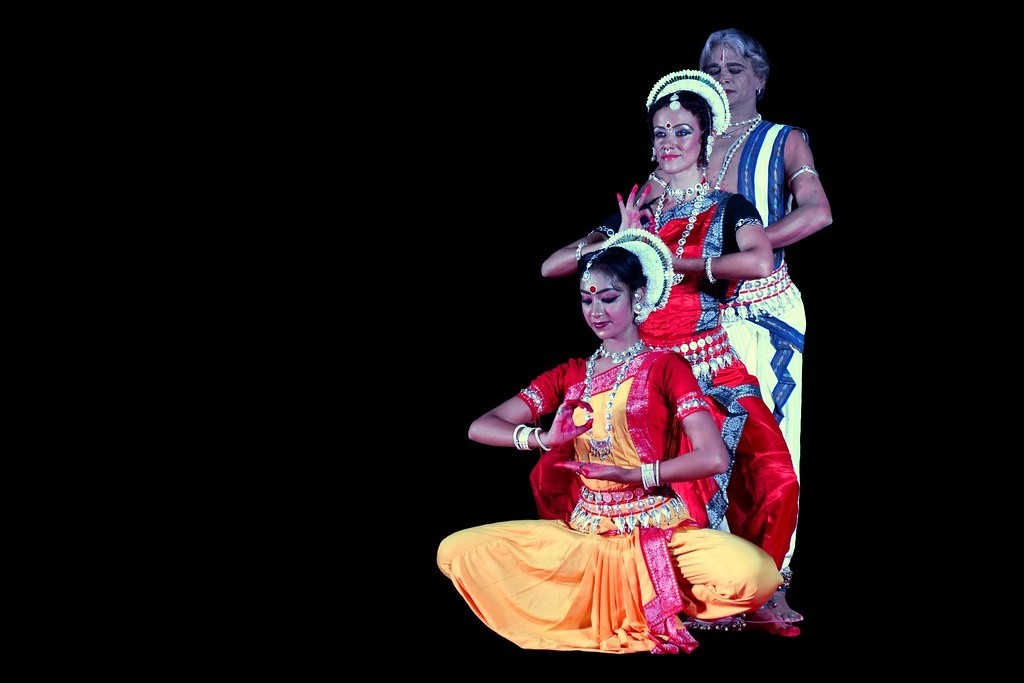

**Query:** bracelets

left=705, top=257, right=716, bottom=284
left=513, top=423, right=551, bottom=452
left=574, top=241, right=588, bottom=260
left=640, top=460, right=660, bottom=490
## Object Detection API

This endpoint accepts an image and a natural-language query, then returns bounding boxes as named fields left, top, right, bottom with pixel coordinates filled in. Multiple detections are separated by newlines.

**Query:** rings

left=557, top=410, right=563, bottom=415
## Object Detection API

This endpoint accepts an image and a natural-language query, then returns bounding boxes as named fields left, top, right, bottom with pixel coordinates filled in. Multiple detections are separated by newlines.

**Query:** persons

left=631, top=27, right=833, bottom=624
left=437, top=228, right=782, bottom=626
left=539, top=68, right=801, bottom=638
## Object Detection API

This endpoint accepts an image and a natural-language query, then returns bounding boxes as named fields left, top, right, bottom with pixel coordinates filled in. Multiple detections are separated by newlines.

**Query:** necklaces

left=711, top=112, right=762, bottom=191
left=581, top=337, right=645, bottom=460
left=645, top=171, right=709, bottom=285
